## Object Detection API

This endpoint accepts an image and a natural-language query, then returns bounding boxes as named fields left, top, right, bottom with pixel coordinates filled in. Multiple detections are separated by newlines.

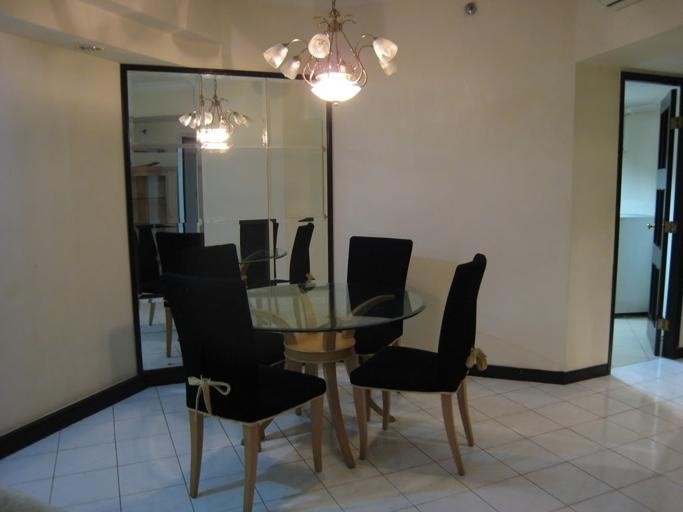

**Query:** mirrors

left=120, top=64, right=335, bottom=373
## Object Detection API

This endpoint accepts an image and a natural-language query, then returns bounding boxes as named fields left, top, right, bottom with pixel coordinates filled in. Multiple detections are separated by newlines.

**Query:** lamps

left=263, top=0, right=398, bottom=103
left=178, top=74, right=252, bottom=151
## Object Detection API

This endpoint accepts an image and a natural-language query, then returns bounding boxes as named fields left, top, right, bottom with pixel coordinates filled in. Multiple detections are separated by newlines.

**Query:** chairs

left=157, top=232, right=204, bottom=358
left=317, top=235, right=413, bottom=421
left=270, top=223, right=314, bottom=286
left=350, top=254, right=487, bottom=475
left=160, top=243, right=326, bottom=512
left=134, top=225, right=161, bottom=325
left=238, top=219, right=278, bottom=288
